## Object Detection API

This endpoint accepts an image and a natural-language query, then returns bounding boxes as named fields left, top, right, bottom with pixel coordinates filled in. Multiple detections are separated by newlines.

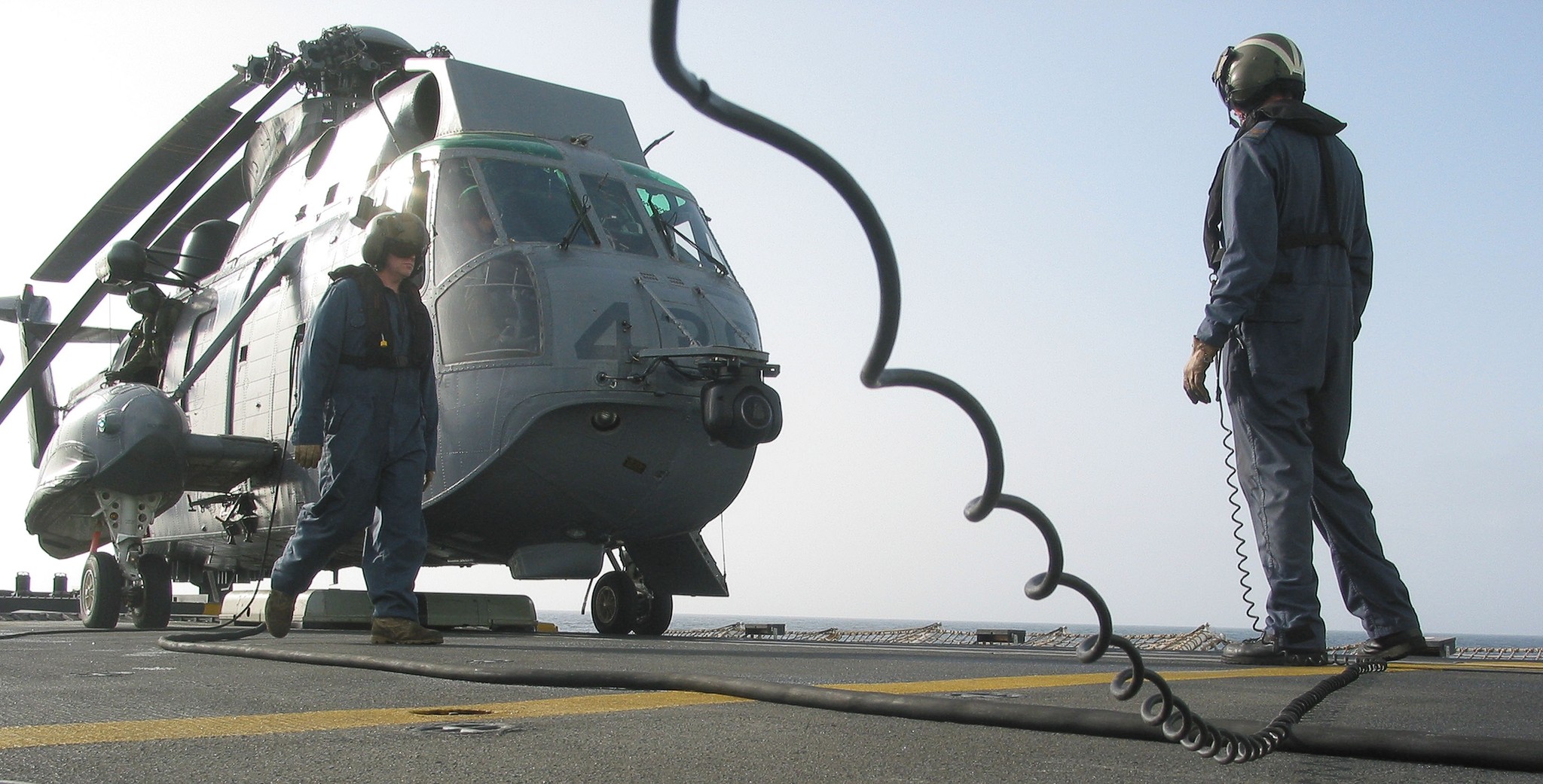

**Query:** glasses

left=387, top=241, right=422, bottom=258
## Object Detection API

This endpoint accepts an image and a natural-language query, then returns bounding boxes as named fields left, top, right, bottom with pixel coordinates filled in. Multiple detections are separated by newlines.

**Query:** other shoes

left=370, top=615, right=444, bottom=644
left=1222, top=632, right=1327, bottom=666
left=264, top=559, right=297, bottom=638
left=1356, top=627, right=1429, bottom=662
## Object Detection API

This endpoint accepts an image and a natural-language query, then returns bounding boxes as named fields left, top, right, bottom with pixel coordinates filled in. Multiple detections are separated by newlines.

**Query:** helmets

left=126, top=283, right=161, bottom=316
left=459, top=183, right=497, bottom=214
left=361, top=212, right=429, bottom=278
left=1211, top=34, right=1305, bottom=104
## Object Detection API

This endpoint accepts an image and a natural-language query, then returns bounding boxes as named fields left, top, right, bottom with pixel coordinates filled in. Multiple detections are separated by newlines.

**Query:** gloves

left=1183, top=335, right=1216, bottom=404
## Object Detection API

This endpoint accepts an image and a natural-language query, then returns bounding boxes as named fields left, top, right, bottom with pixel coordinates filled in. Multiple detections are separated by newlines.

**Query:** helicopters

left=0, top=25, right=784, bottom=637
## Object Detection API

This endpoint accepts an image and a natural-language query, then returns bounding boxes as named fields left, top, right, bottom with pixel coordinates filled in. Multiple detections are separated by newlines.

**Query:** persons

left=265, top=212, right=442, bottom=643
left=1183, top=35, right=1429, bottom=664
